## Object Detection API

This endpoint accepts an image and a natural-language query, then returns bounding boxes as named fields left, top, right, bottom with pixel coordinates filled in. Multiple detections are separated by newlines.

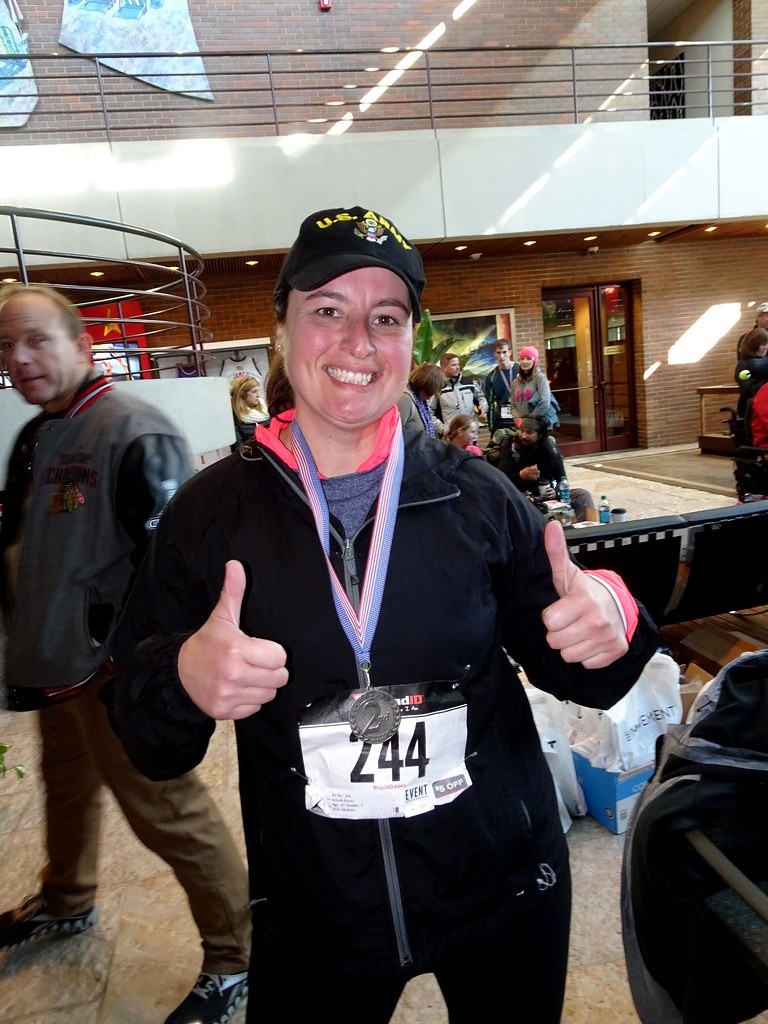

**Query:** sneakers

left=1, top=910, right=97, bottom=953
left=164, top=971, right=251, bottom=1024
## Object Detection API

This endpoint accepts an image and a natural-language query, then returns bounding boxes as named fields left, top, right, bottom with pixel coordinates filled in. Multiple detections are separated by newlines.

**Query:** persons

left=496, top=414, right=595, bottom=522
left=397, top=339, right=553, bottom=460
left=231, top=376, right=271, bottom=453
left=100, top=204, right=659, bottom=1024
left=734, top=301, right=768, bottom=463
left=0, top=285, right=255, bottom=1024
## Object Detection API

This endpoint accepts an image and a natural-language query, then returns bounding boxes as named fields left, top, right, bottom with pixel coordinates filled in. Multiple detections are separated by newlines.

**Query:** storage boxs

left=573, top=738, right=656, bottom=835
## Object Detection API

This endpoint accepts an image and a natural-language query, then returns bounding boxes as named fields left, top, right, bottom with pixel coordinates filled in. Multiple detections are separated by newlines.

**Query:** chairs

left=620, top=653, right=768, bottom=1023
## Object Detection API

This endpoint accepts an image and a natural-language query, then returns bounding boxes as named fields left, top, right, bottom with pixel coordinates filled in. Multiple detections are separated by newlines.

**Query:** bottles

left=598, top=496, right=610, bottom=523
left=558, top=476, right=571, bottom=505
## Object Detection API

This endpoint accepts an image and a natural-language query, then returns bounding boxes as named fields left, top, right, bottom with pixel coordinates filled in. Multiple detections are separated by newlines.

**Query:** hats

left=755, top=302, right=768, bottom=322
left=518, top=346, right=539, bottom=367
left=275, top=206, right=426, bottom=323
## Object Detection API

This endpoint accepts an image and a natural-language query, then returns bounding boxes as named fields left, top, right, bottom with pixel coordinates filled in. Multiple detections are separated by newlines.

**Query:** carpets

left=571, top=449, right=738, bottom=499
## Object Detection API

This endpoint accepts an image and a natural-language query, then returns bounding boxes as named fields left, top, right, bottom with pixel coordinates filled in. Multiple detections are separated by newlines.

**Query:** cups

left=538, top=480, right=550, bottom=496
left=611, top=508, right=627, bottom=524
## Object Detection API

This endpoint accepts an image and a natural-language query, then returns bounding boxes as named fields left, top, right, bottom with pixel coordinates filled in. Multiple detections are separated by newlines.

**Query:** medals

left=348, top=690, right=399, bottom=744
